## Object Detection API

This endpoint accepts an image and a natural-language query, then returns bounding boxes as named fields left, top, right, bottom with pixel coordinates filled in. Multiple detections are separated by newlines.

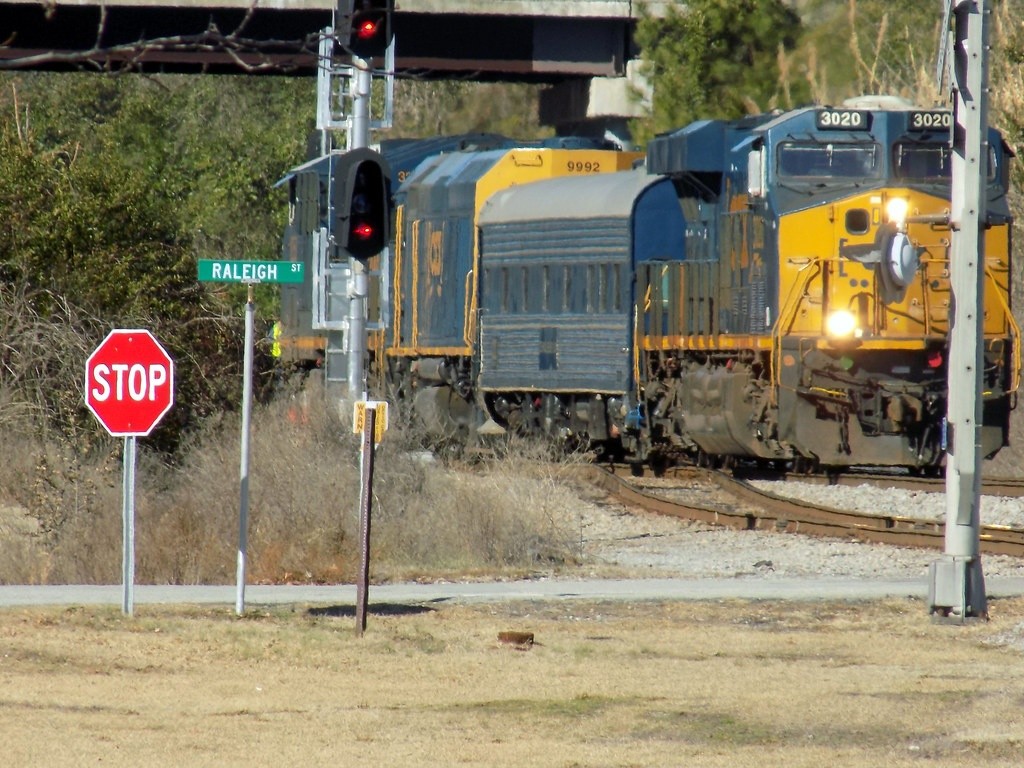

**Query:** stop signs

left=81, top=330, right=177, bottom=439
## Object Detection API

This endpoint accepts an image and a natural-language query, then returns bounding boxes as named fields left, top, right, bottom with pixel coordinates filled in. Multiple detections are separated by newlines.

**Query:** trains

left=267, top=89, right=1023, bottom=483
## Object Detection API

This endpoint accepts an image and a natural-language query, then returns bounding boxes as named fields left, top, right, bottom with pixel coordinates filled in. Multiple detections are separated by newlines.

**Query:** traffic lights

left=334, top=0, right=397, bottom=63
left=330, top=148, right=393, bottom=261
